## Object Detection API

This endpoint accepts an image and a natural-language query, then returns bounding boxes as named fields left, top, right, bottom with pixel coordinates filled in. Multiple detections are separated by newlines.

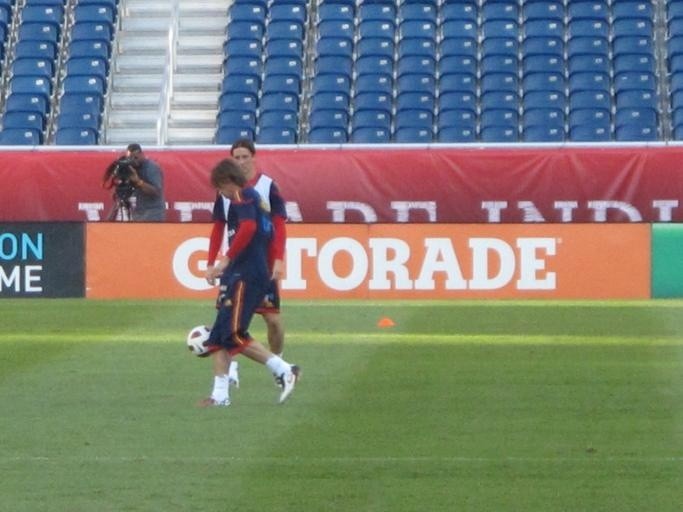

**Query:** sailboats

left=188, top=326, right=211, bottom=357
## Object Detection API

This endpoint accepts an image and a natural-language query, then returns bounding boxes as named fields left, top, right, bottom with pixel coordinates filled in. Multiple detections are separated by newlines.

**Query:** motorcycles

left=109, top=200, right=133, bottom=221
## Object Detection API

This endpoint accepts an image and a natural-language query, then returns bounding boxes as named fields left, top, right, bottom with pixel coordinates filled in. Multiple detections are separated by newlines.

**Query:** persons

left=203, top=138, right=288, bottom=390
left=193, top=155, right=303, bottom=407
left=102, top=142, right=167, bottom=222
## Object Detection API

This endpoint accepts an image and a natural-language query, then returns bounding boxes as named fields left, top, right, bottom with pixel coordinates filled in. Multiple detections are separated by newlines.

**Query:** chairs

left=0, top=0, right=122, bottom=142
left=214, top=1, right=682, bottom=143
left=193, top=397, right=233, bottom=409
left=276, top=364, right=302, bottom=404
